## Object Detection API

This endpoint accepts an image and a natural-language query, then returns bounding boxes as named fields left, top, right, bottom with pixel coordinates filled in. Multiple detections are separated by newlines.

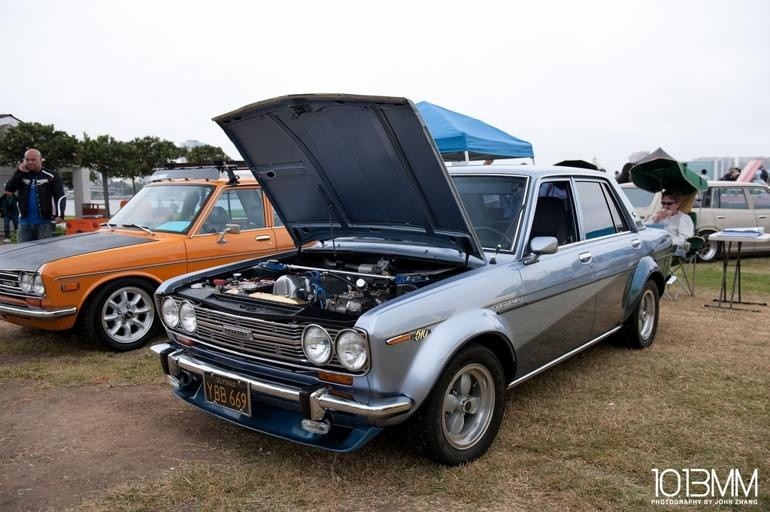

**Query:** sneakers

left=3, top=238, right=11, bottom=243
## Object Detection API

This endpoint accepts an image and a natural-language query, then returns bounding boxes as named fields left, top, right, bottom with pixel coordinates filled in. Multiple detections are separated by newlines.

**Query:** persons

left=5, top=149, right=66, bottom=242
left=719, top=166, right=768, bottom=183
left=0, top=191, right=19, bottom=243
left=644, top=190, right=695, bottom=261
left=616, top=163, right=636, bottom=184
left=699, top=169, right=711, bottom=180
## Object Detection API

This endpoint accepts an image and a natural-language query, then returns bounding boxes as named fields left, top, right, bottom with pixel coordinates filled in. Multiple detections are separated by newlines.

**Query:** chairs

left=463, top=193, right=487, bottom=226
left=242, top=207, right=263, bottom=229
left=201, top=207, right=229, bottom=233
left=532, top=196, right=568, bottom=246
left=653, top=213, right=706, bottom=302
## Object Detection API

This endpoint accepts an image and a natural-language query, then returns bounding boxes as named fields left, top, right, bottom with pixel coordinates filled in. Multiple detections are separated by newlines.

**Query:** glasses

left=661, top=201, right=675, bottom=206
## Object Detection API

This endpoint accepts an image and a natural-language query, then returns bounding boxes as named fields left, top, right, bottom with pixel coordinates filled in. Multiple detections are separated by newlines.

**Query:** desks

left=704, top=228, right=770, bottom=312
